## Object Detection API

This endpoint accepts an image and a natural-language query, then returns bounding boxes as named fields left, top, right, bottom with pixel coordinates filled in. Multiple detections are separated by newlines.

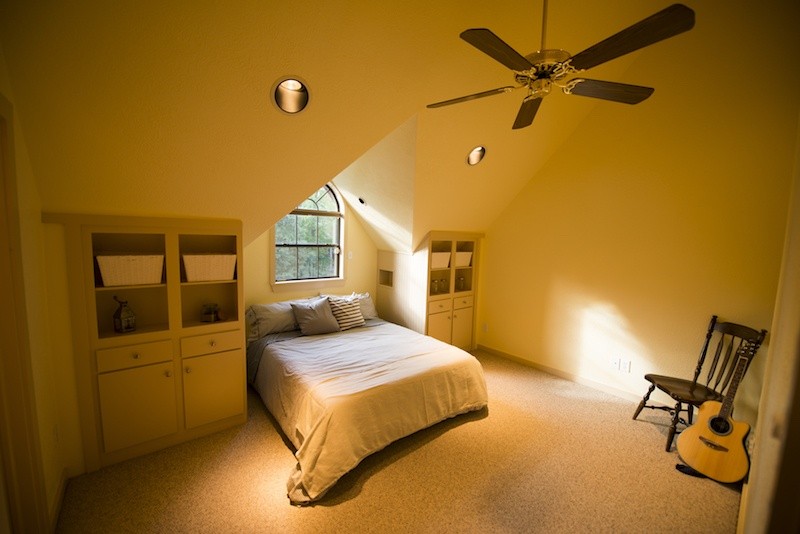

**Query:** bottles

left=440, top=279, right=449, bottom=291
left=458, top=277, right=464, bottom=290
left=431, top=280, right=439, bottom=295
left=199, top=304, right=219, bottom=321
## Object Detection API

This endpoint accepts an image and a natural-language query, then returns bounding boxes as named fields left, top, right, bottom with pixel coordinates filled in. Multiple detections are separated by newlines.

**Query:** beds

left=248, top=318, right=488, bottom=506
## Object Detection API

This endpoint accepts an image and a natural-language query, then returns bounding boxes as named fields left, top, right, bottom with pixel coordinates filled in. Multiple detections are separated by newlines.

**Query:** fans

left=426, top=0, right=696, bottom=129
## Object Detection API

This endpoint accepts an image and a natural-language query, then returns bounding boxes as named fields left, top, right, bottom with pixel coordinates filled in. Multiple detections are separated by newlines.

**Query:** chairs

left=633, top=314, right=767, bottom=453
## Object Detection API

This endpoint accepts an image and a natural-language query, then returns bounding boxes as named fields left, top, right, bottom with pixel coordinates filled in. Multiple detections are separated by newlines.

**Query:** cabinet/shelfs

left=81, top=224, right=247, bottom=465
left=426, top=237, right=480, bottom=351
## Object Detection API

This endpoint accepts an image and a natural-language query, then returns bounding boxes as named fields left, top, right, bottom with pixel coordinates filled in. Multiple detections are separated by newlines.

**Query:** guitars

left=675, top=340, right=761, bottom=483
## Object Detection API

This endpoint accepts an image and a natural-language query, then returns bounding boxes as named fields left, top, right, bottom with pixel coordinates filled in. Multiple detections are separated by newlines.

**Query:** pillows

left=252, top=292, right=377, bottom=337
